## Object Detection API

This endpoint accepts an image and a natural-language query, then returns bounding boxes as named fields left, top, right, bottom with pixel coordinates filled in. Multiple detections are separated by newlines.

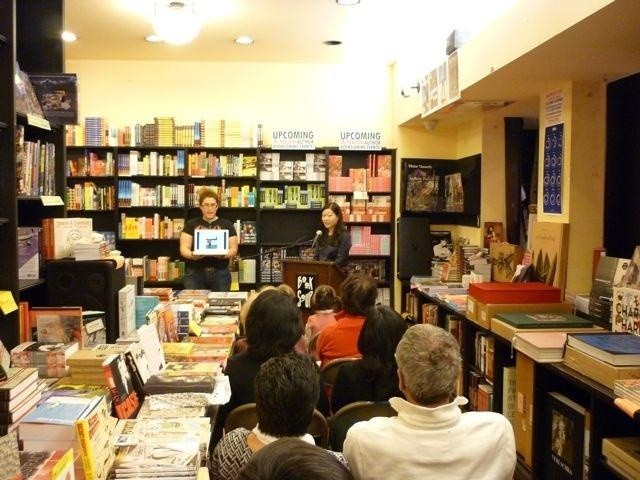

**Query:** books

left=407, top=226, right=640, bottom=479
left=68, top=115, right=396, bottom=300
left=6, top=69, right=121, bottom=311
left=1, top=277, right=265, bottom=480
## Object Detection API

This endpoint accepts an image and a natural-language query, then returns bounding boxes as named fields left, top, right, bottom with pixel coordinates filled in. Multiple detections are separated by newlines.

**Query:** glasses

left=199, top=202, right=217, bottom=208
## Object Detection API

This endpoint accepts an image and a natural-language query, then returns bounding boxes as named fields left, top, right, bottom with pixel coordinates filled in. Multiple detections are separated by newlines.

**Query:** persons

left=235, top=443, right=356, bottom=480
left=332, top=309, right=423, bottom=416
left=181, top=186, right=239, bottom=293
left=241, top=270, right=413, bottom=364
left=343, top=321, right=519, bottom=479
left=211, top=356, right=353, bottom=480
left=313, top=201, right=353, bottom=269
left=229, top=301, right=331, bottom=422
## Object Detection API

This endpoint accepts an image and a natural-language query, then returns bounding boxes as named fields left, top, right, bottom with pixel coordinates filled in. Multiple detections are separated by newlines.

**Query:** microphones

left=310, top=229, right=323, bottom=248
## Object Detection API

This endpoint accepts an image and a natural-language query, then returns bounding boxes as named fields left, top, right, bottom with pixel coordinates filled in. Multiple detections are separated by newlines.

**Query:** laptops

left=193, top=230, right=229, bottom=256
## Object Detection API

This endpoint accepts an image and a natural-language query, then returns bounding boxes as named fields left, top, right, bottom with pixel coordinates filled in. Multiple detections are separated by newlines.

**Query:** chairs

left=224, top=403, right=328, bottom=451
left=319, top=357, right=360, bottom=384
left=328, top=400, right=469, bottom=452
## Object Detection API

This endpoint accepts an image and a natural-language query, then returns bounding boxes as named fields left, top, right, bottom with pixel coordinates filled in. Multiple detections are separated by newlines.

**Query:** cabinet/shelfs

left=405, top=277, right=639, bottom=479
left=11, top=115, right=63, bottom=289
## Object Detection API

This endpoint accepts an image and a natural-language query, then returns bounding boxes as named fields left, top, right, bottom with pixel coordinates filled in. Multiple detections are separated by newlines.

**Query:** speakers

left=46, top=258, right=126, bottom=344
left=398, top=217, right=434, bottom=280
